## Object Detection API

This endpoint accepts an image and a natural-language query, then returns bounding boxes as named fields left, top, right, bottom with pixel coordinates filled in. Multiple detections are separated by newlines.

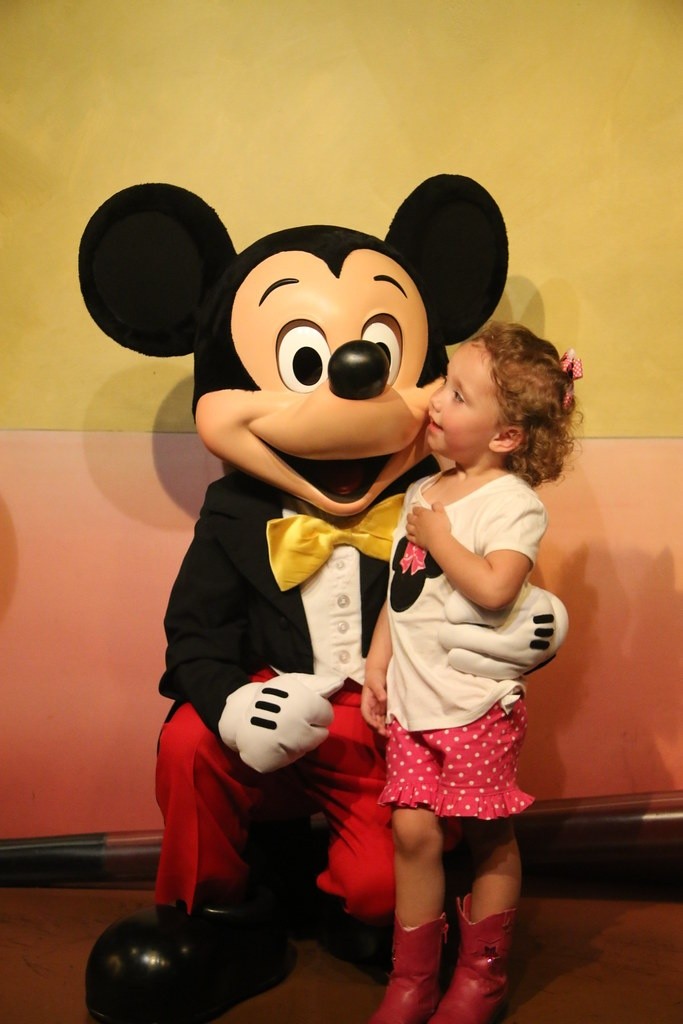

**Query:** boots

left=364, top=909, right=450, bottom=1024
left=425, top=892, right=514, bottom=1024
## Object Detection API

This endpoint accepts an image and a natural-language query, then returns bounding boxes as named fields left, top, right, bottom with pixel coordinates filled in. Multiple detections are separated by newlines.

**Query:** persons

left=360, top=324, right=581, bottom=1024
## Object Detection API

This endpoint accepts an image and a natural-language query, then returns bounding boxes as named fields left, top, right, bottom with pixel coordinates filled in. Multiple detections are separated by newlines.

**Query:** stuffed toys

left=78, top=173, right=567, bottom=1024
